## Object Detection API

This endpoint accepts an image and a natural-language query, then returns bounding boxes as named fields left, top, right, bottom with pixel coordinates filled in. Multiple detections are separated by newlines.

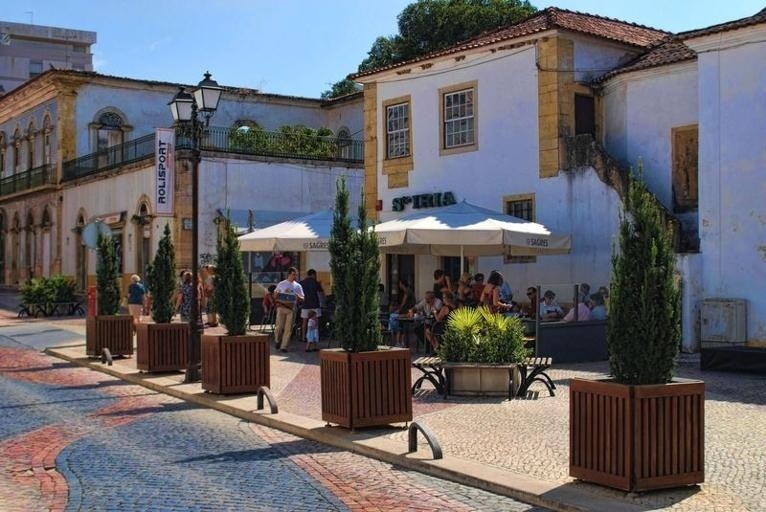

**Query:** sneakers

left=313, top=348, right=320, bottom=352
left=280, top=348, right=289, bottom=353
left=275, top=341, right=281, bottom=350
left=306, top=349, right=312, bottom=352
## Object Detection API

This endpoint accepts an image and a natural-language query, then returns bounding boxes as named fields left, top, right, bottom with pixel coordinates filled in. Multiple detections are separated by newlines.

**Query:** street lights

left=167, top=69, right=224, bottom=388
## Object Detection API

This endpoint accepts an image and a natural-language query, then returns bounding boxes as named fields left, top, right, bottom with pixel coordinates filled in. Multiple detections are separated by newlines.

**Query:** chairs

left=251, top=291, right=540, bottom=355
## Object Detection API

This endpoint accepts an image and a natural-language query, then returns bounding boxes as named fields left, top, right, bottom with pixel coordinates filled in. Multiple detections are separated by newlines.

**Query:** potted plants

left=16, top=274, right=79, bottom=316
left=439, top=304, right=526, bottom=398
left=314, top=170, right=413, bottom=429
left=134, top=220, right=188, bottom=375
left=84, top=229, right=132, bottom=357
left=568, top=149, right=706, bottom=492
left=198, top=206, right=271, bottom=396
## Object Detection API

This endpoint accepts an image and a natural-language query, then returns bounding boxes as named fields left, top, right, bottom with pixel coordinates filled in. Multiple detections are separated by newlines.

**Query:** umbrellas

left=237, top=209, right=373, bottom=253
left=355, top=199, right=572, bottom=284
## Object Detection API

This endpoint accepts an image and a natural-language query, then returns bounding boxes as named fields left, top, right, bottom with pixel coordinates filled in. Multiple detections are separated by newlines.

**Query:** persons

left=128, top=268, right=152, bottom=336
left=539, top=283, right=607, bottom=322
left=377, top=281, right=454, bottom=352
left=433, top=268, right=537, bottom=323
left=262, top=268, right=327, bottom=352
left=171, top=267, right=218, bottom=328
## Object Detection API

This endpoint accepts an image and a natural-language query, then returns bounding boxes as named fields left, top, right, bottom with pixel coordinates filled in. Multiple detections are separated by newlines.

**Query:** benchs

left=16, top=297, right=84, bottom=319
left=517, top=357, right=555, bottom=396
left=410, top=356, right=441, bottom=397
